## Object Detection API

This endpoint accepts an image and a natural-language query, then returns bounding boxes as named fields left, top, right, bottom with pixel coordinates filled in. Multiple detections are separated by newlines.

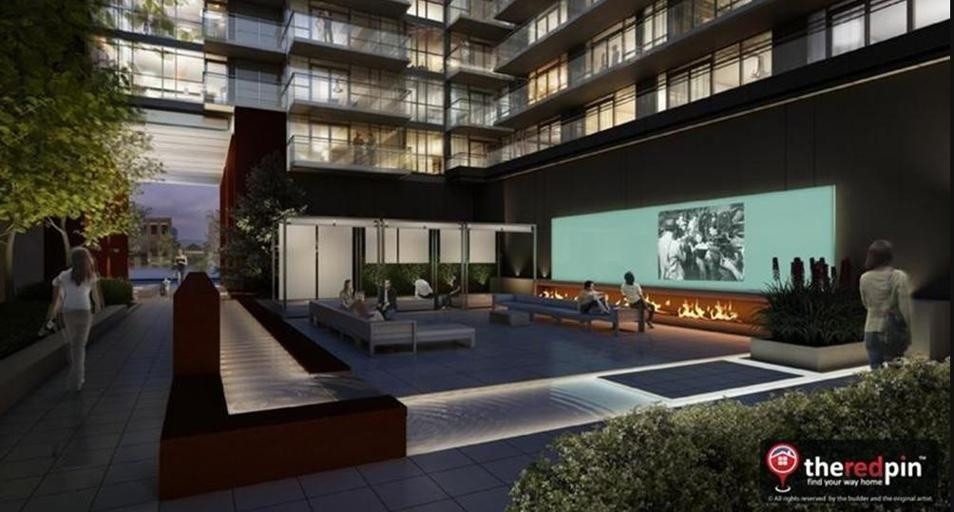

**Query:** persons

left=619, top=271, right=655, bottom=333
left=173, top=246, right=188, bottom=288
left=659, top=211, right=742, bottom=281
left=858, top=238, right=915, bottom=369
left=376, top=277, right=398, bottom=321
left=45, top=247, right=104, bottom=392
left=578, top=279, right=611, bottom=315
left=314, top=12, right=324, bottom=44
left=365, top=132, right=379, bottom=168
left=352, top=131, right=363, bottom=166
left=414, top=274, right=441, bottom=299
left=441, top=273, right=460, bottom=310
left=610, top=45, right=620, bottom=66
left=339, top=278, right=374, bottom=320
left=323, top=10, right=333, bottom=45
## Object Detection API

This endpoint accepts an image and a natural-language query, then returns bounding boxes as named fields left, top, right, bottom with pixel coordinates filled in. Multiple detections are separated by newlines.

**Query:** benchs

left=621, top=306, right=655, bottom=323
left=395, top=293, right=493, bottom=311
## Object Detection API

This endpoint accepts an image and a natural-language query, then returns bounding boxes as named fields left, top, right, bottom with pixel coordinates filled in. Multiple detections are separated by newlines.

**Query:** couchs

left=492, top=293, right=620, bottom=330
left=309, top=297, right=477, bottom=357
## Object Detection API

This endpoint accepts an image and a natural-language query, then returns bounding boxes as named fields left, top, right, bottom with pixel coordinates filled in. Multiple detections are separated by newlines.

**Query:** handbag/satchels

left=881, top=301, right=913, bottom=347
